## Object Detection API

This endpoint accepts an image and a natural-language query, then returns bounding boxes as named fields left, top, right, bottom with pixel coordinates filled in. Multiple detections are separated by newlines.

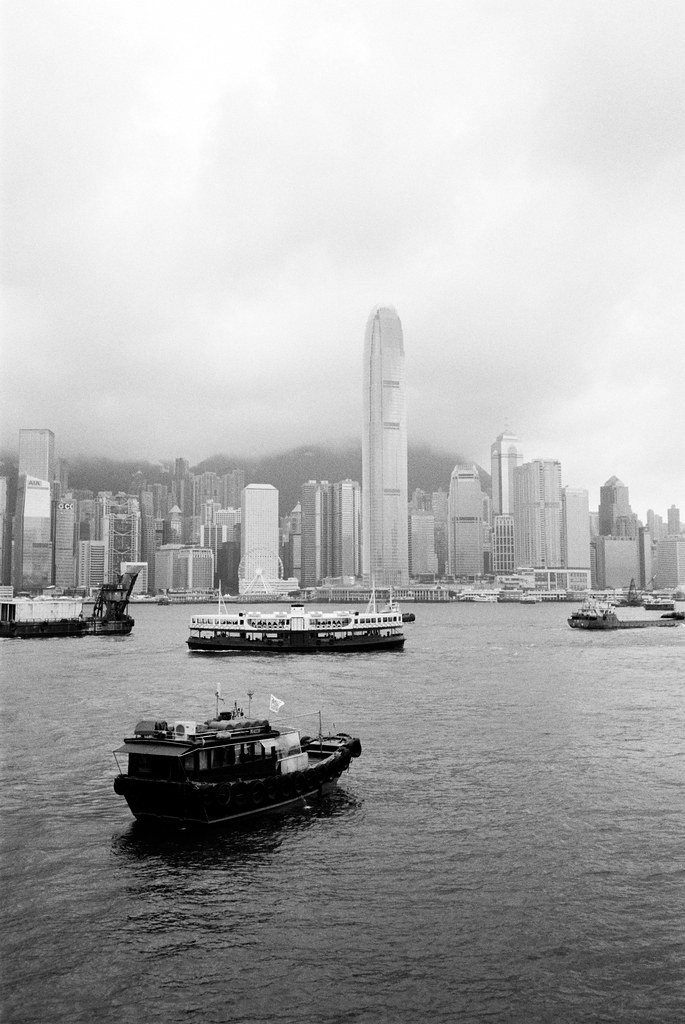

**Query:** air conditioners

left=174, top=721, right=195, bottom=740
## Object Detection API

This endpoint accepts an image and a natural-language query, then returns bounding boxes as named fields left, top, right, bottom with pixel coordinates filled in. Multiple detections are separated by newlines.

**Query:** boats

left=567, top=595, right=624, bottom=630
left=461, top=576, right=685, bottom=619
left=187, top=611, right=416, bottom=656
left=113, top=678, right=362, bottom=835
left=0, top=560, right=149, bottom=638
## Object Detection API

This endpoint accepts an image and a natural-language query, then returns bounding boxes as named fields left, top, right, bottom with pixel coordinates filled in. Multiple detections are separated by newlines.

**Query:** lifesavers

left=266, top=639, right=273, bottom=645
left=213, top=783, right=232, bottom=810
left=40, top=622, right=49, bottom=631
left=251, top=781, right=266, bottom=807
left=316, top=640, right=321, bottom=645
left=232, top=782, right=250, bottom=811
left=80, top=622, right=87, bottom=629
left=10, top=623, right=17, bottom=631
left=278, top=641, right=284, bottom=646
left=329, top=638, right=335, bottom=645
left=102, top=619, right=109, bottom=626
left=264, top=749, right=352, bottom=803
left=126, top=621, right=132, bottom=627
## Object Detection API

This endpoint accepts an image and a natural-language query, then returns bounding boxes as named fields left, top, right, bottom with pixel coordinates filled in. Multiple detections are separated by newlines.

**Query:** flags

left=269, top=694, right=285, bottom=713
